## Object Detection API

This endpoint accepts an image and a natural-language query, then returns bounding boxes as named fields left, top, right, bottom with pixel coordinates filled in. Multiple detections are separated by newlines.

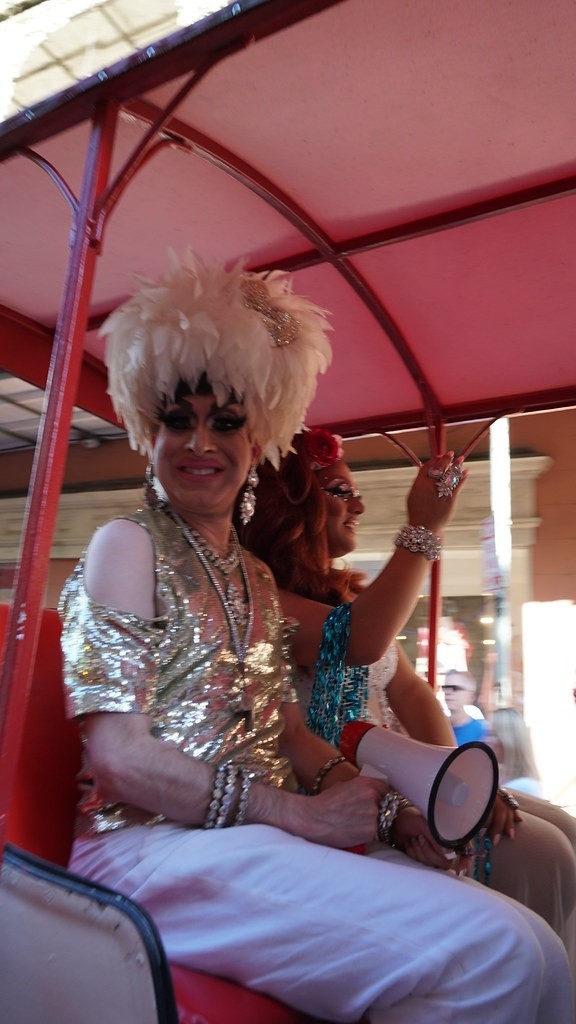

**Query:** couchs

left=0, top=605, right=308, bottom=1024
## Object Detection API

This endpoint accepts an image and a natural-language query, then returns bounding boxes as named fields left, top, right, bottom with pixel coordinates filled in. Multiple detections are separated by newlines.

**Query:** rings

left=503, top=795, right=519, bottom=809
left=427, top=466, right=443, bottom=478
left=418, top=834, right=425, bottom=845
left=434, top=461, right=469, bottom=498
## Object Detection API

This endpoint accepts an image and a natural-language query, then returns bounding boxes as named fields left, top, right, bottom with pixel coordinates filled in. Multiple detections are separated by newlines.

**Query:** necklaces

left=170, top=508, right=255, bottom=733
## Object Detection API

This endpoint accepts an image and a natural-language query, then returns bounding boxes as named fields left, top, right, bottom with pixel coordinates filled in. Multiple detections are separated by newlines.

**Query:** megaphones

left=339, top=721, right=499, bottom=857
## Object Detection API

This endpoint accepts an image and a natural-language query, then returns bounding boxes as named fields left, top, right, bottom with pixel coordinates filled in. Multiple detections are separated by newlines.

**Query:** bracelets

left=394, top=524, right=443, bottom=562
left=204, top=763, right=253, bottom=828
left=310, top=755, right=345, bottom=794
left=376, top=791, right=410, bottom=847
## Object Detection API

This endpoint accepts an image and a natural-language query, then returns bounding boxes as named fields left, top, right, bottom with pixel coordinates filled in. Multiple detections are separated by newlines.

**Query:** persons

left=233, top=428, right=576, bottom=931
left=481, top=707, right=542, bottom=799
left=441, top=669, right=485, bottom=748
left=55, top=241, right=575, bottom=1024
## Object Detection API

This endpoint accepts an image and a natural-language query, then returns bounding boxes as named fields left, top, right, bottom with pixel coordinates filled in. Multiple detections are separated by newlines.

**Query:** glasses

left=321, top=479, right=362, bottom=502
